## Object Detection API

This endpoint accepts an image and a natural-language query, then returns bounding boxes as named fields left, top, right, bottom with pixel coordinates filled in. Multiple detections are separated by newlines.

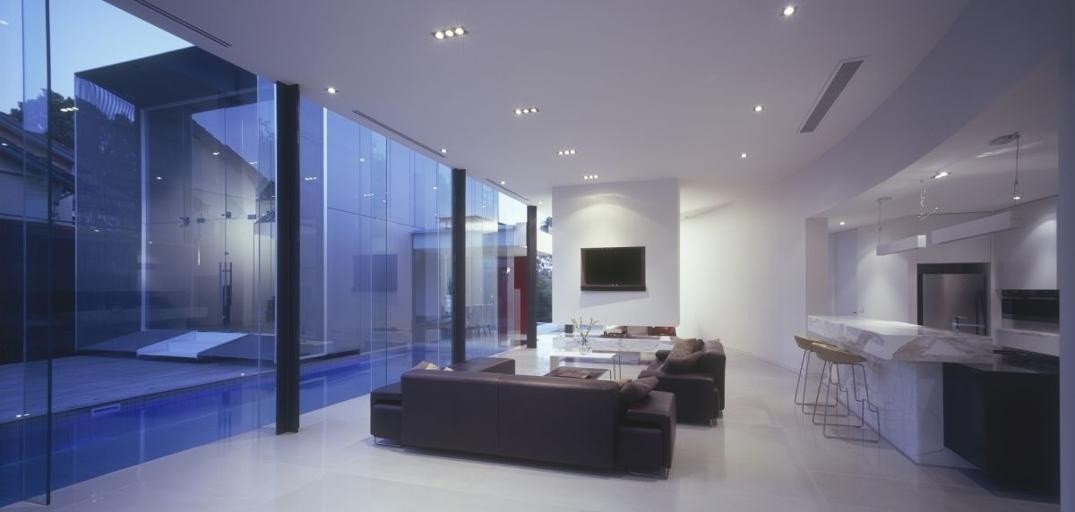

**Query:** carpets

left=543, top=365, right=609, bottom=379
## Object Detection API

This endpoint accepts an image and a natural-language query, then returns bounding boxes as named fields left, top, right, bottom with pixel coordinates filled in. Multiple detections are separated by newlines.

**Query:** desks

left=550, top=351, right=622, bottom=381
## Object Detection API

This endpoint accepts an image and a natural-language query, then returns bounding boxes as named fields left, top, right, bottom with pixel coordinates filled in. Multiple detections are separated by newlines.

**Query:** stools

left=793, top=335, right=850, bottom=418
left=811, top=342, right=882, bottom=443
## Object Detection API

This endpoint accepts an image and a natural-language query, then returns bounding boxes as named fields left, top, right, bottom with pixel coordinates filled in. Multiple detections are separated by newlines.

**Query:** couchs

left=638, top=339, right=725, bottom=423
left=401, top=369, right=677, bottom=481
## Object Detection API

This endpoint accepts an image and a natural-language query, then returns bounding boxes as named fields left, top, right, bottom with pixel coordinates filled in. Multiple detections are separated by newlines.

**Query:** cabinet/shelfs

left=942, top=349, right=1060, bottom=498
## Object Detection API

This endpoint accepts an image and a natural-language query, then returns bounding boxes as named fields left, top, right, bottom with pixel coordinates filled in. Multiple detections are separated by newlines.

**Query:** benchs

left=369, top=356, right=516, bottom=448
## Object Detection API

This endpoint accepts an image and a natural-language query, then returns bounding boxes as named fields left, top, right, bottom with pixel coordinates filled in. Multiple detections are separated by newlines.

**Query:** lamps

left=989, top=131, right=1023, bottom=201
left=917, top=170, right=951, bottom=224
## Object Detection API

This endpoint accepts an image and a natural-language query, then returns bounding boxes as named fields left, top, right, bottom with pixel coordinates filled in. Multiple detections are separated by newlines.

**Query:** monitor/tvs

left=580, top=245, right=646, bottom=292
